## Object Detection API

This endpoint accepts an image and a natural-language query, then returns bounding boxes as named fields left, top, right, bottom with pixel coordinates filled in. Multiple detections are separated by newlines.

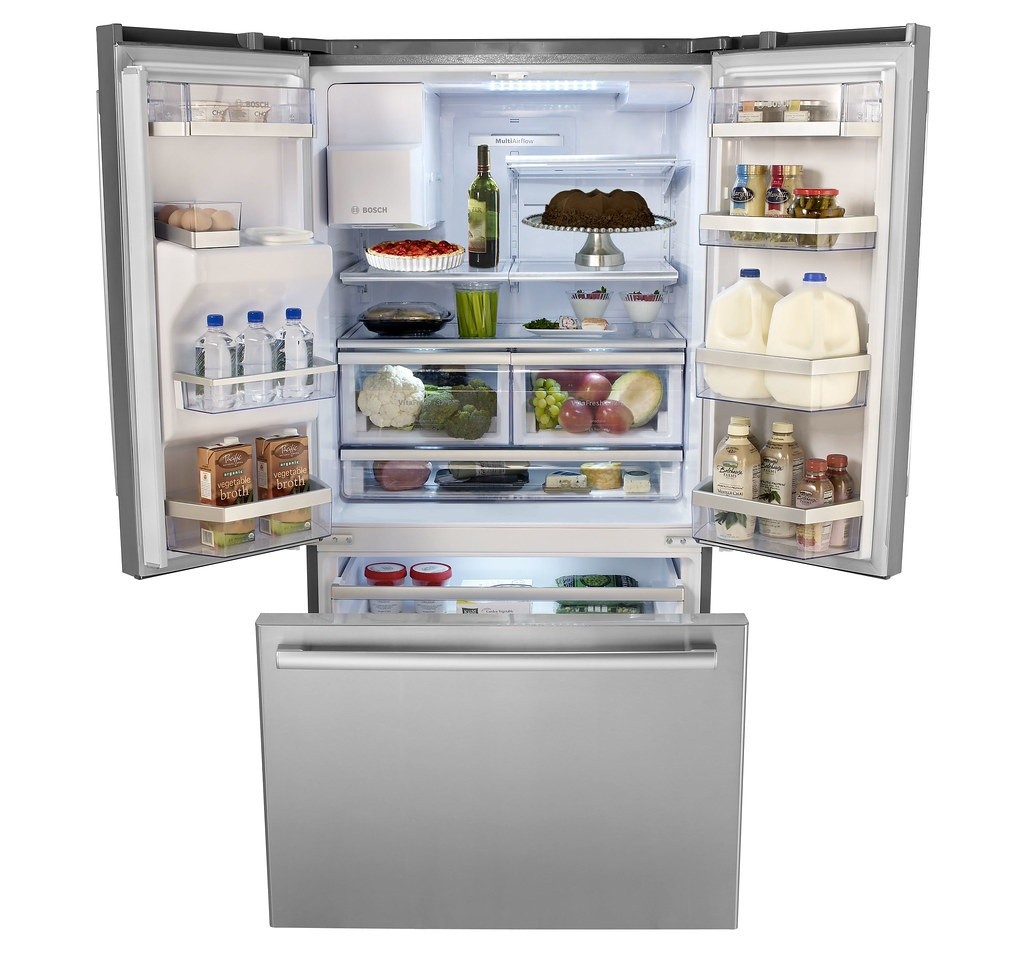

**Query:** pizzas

left=367, top=238, right=466, bottom=257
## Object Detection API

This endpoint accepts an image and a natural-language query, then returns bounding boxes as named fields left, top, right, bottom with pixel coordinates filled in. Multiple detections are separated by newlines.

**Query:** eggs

left=157, top=204, right=235, bottom=232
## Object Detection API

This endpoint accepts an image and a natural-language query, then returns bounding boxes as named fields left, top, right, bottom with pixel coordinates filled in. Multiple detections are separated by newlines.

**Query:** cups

left=452, top=281, right=502, bottom=337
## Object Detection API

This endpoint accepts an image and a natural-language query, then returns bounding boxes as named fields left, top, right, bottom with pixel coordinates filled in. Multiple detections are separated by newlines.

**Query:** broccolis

left=357, top=365, right=498, bottom=441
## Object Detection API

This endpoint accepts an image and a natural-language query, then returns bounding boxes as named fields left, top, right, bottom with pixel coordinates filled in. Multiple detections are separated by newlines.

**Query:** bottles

left=717, top=416, right=759, bottom=453
left=825, top=454, right=854, bottom=548
left=194, top=313, right=236, bottom=411
left=766, top=164, right=805, bottom=246
left=235, top=310, right=276, bottom=407
left=275, top=308, right=313, bottom=399
left=763, top=272, right=860, bottom=409
left=728, top=164, right=769, bottom=241
left=796, top=458, right=834, bottom=554
left=759, top=422, right=804, bottom=538
left=713, top=425, right=761, bottom=542
left=702, top=270, right=785, bottom=400
left=467, top=145, right=499, bottom=269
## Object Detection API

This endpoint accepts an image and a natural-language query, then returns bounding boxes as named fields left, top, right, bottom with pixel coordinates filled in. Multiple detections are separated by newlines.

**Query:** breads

left=579, top=462, right=622, bottom=489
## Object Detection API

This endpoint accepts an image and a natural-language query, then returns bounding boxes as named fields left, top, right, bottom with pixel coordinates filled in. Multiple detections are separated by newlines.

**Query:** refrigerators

left=94, top=21, right=932, bottom=929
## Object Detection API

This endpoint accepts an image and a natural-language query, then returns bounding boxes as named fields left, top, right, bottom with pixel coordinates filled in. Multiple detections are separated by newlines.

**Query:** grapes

left=530, top=377, right=571, bottom=430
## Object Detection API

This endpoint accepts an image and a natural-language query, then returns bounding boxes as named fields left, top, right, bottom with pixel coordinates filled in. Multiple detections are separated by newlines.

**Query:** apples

left=541, top=372, right=634, bottom=431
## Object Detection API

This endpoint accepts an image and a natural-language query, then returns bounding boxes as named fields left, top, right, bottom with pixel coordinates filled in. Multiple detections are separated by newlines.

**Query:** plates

left=523, top=320, right=618, bottom=338
left=357, top=301, right=455, bottom=335
left=244, top=226, right=313, bottom=247
left=434, top=469, right=529, bottom=491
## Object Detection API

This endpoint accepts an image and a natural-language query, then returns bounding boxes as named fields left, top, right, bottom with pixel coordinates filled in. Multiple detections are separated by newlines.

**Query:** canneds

left=728, top=163, right=844, bottom=249
left=735, top=100, right=827, bottom=123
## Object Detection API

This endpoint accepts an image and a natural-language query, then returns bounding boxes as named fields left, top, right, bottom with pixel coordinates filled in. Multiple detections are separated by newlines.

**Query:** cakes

left=542, top=188, right=655, bottom=228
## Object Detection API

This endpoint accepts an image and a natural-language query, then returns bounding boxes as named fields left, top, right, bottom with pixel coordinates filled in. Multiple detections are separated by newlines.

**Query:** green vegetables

left=522, top=318, right=558, bottom=329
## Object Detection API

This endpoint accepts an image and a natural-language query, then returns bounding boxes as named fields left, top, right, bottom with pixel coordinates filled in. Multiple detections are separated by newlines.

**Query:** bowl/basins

left=565, top=289, right=614, bottom=320
left=618, top=291, right=669, bottom=323
